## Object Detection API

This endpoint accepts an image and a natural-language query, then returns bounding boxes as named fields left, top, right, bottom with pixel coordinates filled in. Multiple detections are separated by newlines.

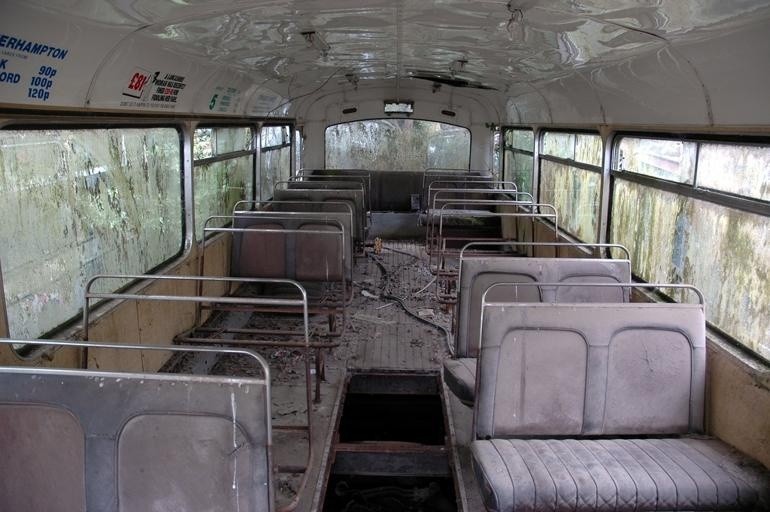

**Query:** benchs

left=0, top=170, right=770, bottom=511
